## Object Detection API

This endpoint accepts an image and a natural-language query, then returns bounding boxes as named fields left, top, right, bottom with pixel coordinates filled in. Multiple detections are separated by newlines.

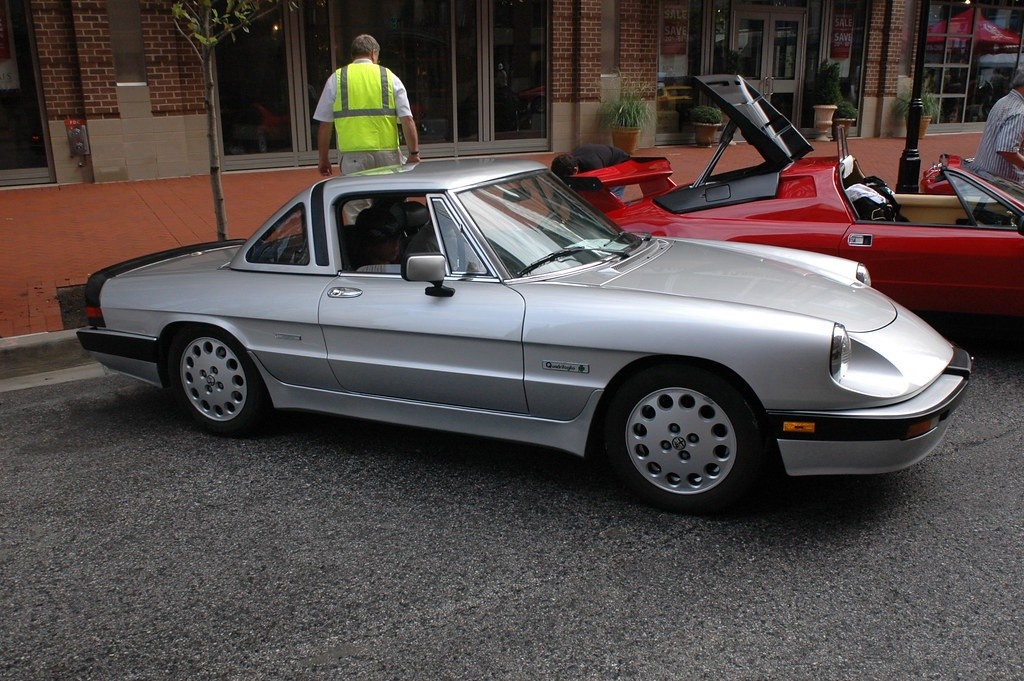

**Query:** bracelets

left=410, top=151, right=418, bottom=156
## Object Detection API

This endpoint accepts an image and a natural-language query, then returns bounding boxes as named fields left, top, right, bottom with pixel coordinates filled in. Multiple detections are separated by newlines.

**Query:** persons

left=973, top=73, right=1024, bottom=185
left=312, top=34, right=420, bottom=223
left=550, top=144, right=631, bottom=199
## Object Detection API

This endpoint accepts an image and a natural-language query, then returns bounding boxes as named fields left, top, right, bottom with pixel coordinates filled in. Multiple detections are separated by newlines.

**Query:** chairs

left=850, top=177, right=912, bottom=224
left=400, top=201, right=429, bottom=248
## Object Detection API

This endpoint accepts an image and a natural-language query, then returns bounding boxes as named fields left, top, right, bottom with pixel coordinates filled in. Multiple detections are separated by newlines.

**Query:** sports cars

left=568, top=74, right=1024, bottom=317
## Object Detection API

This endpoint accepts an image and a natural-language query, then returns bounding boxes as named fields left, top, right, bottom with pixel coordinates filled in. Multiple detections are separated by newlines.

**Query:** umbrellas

left=926, top=10, right=1020, bottom=45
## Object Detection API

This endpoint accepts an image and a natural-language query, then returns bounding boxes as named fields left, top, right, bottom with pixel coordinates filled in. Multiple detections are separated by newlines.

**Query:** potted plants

left=830, top=101, right=858, bottom=140
left=812, top=60, right=841, bottom=142
left=897, top=79, right=938, bottom=139
left=594, top=67, right=657, bottom=156
left=691, top=106, right=722, bottom=148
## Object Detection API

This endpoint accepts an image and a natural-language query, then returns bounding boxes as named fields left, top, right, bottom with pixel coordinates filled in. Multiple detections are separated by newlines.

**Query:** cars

left=77, top=159, right=971, bottom=515
